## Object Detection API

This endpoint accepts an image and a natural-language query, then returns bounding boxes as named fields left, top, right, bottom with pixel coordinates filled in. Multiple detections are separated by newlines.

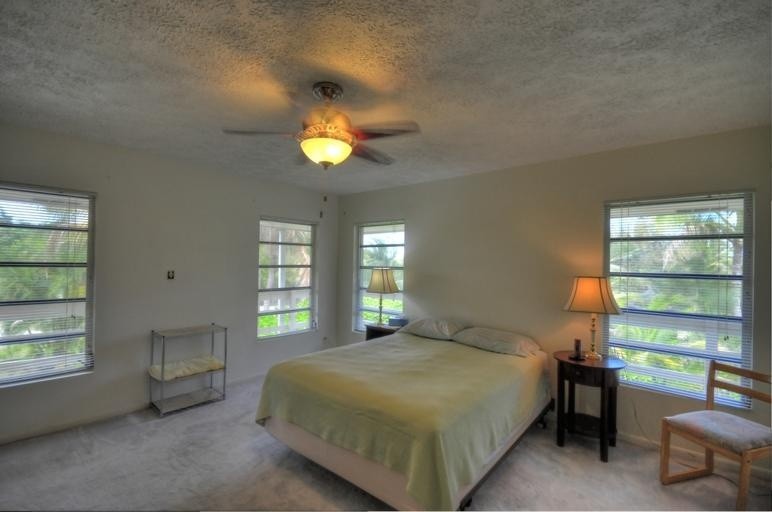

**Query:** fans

left=219, top=82, right=420, bottom=220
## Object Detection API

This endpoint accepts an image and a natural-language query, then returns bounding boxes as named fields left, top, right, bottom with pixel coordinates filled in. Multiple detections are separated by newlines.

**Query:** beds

left=254, top=331, right=553, bottom=512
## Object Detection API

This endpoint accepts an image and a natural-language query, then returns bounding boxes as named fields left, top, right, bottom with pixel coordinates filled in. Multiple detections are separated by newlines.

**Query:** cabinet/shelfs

left=147, top=322, right=228, bottom=419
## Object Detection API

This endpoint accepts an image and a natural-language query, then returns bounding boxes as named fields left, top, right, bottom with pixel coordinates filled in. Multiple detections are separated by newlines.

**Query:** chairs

left=659, top=359, right=772, bottom=511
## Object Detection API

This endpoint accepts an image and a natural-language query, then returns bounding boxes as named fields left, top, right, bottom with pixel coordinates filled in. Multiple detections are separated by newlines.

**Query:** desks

left=552, top=350, right=629, bottom=462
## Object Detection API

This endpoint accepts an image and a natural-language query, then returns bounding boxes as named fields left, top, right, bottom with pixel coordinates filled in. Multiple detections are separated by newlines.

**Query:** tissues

left=389, top=312, right=408, bottom=326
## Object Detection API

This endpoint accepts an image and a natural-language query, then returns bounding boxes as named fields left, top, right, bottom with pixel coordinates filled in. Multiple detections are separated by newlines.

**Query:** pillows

left=398, top=315, right=541, bottom=358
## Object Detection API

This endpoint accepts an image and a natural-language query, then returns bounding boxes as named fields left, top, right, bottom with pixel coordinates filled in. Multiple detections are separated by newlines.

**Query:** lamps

left=366, top=266, right=400, bottom=325
left=562, top=275, right=622, bottom=362
left=298, top=132, right=354, bottom=171
